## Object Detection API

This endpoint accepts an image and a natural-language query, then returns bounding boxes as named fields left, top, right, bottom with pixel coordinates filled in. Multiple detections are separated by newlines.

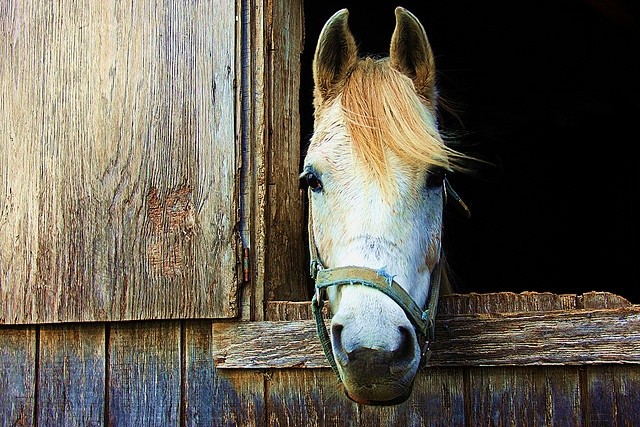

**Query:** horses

left=298, top=4, right=498, bottom=409
